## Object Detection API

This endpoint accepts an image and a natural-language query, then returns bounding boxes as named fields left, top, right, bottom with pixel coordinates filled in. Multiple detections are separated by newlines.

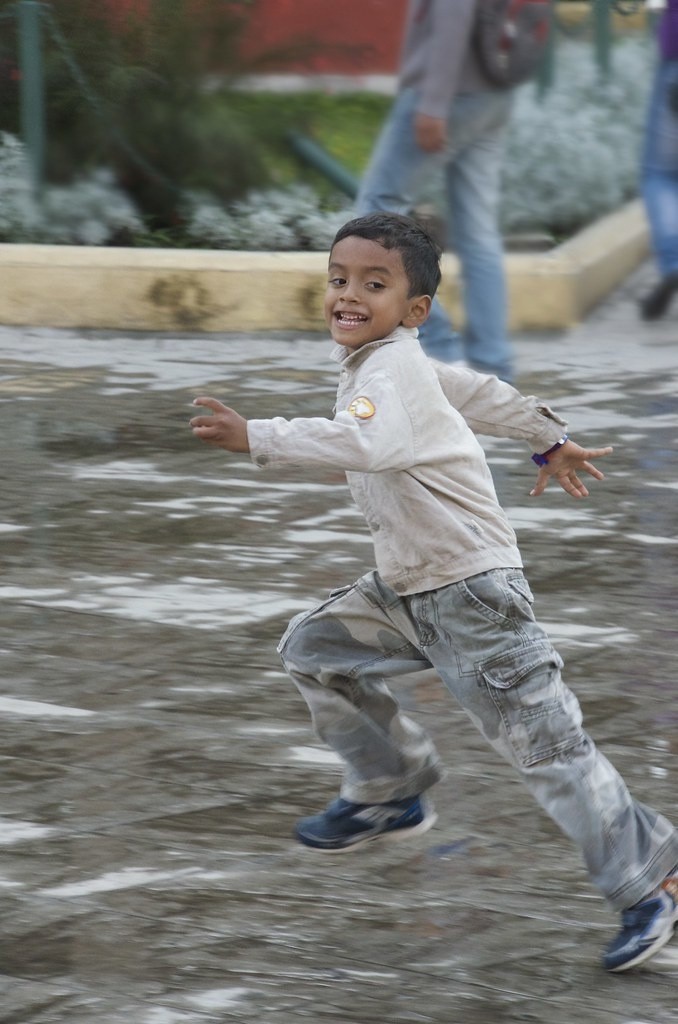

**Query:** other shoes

left=639, top=271, right=678, bottom=318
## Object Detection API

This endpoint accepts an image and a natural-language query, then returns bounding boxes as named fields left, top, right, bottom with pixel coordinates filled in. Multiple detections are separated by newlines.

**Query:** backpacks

left=472, top=0, right=551, bottom=90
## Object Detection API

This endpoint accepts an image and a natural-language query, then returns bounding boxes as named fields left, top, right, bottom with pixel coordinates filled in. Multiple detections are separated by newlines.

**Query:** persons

left=642, top=0, right=678, bottom=320
left=190, top=213, right=678, bottom=972
left=352, top=0, right=519, bottom=389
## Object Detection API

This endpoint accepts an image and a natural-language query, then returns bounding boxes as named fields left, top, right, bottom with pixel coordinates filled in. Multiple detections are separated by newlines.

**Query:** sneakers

left=602, top=865, right=678, bottom=973
left=294, top=790, right=438, bottom=854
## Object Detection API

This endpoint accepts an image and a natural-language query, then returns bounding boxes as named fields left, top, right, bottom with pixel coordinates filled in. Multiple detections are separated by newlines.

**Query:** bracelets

left=531, top=435, right=568, bottom=467
